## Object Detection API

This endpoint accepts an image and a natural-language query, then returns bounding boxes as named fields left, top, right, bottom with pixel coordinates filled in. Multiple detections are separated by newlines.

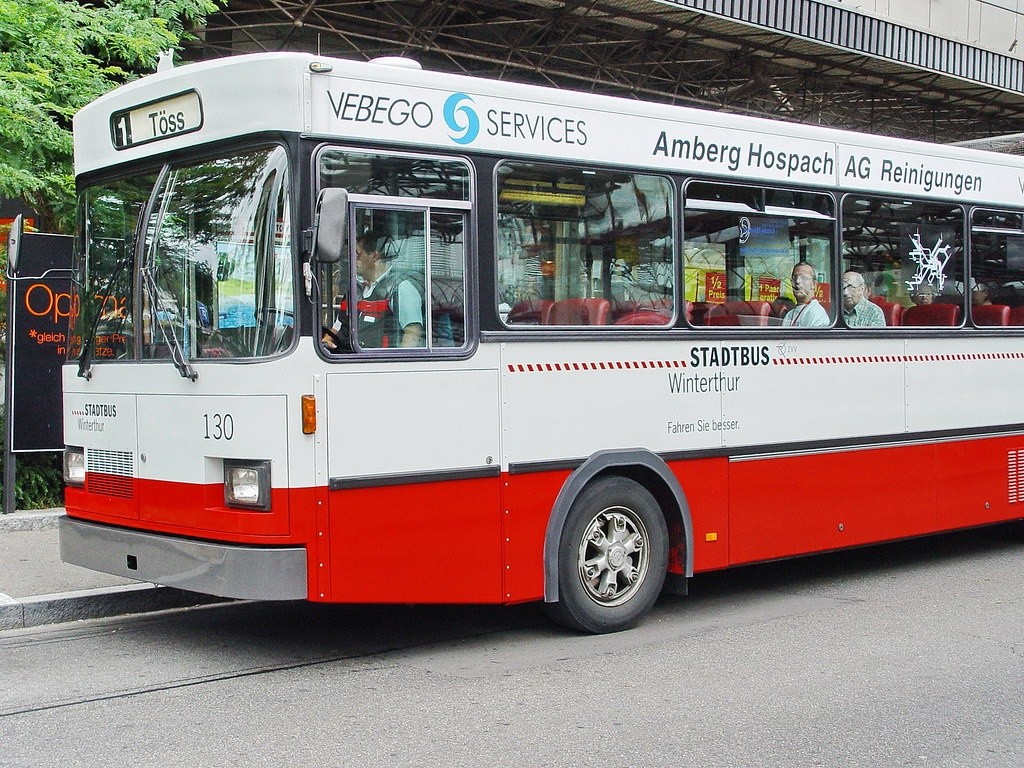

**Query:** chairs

left=505, top=295, right=1024, bottom=327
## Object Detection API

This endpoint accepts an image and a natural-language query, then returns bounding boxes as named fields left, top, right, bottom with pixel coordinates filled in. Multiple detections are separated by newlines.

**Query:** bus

left=4, top=44, right=1024, bottom=639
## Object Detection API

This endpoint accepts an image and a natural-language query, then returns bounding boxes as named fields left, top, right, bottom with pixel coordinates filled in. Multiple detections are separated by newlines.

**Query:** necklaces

left=789, top=297, right=815, bottom=326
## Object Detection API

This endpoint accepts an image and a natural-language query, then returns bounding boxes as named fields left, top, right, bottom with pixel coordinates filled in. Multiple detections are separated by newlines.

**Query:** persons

left=909, top=279, right=938, bottom=306
left=321, top=229, right=425, bottom=356
left=782, top=261, right=832, bottom=327
left=841, top=271, right=888, bottom=328
left=972, top=278, right=995, bottom=304
